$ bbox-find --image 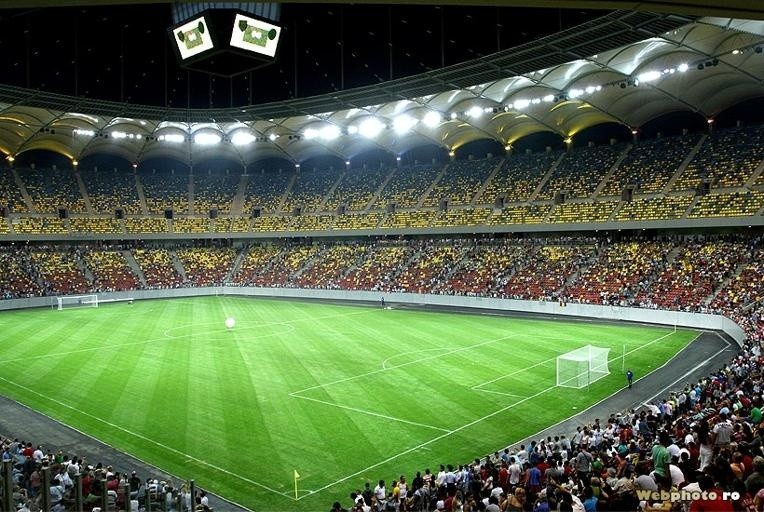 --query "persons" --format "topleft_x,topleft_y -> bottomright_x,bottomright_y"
0,124 -> 764,512
